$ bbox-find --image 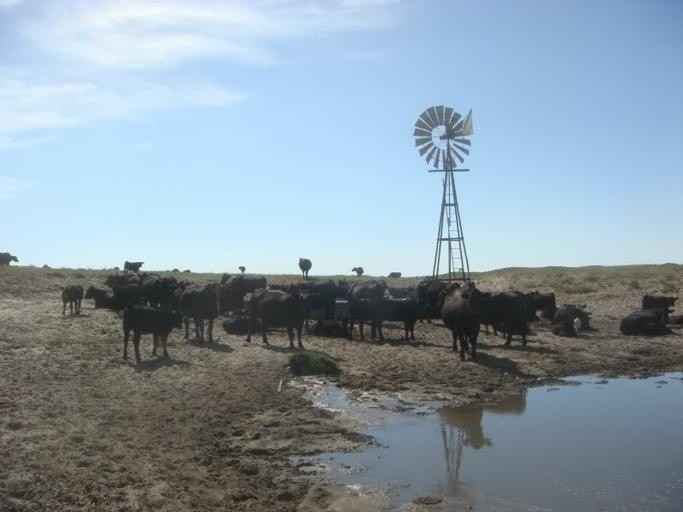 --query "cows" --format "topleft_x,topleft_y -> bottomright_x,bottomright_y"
83,272 -> 600,365
238,265 -> 245,273
299,257 -> 312,281
642,294 -> 679,311
124,261 -> 145,275
0,253 -> 19,266
619,308 -> 675,336
60,285 -> 84,315
352,267 -> 364,277
